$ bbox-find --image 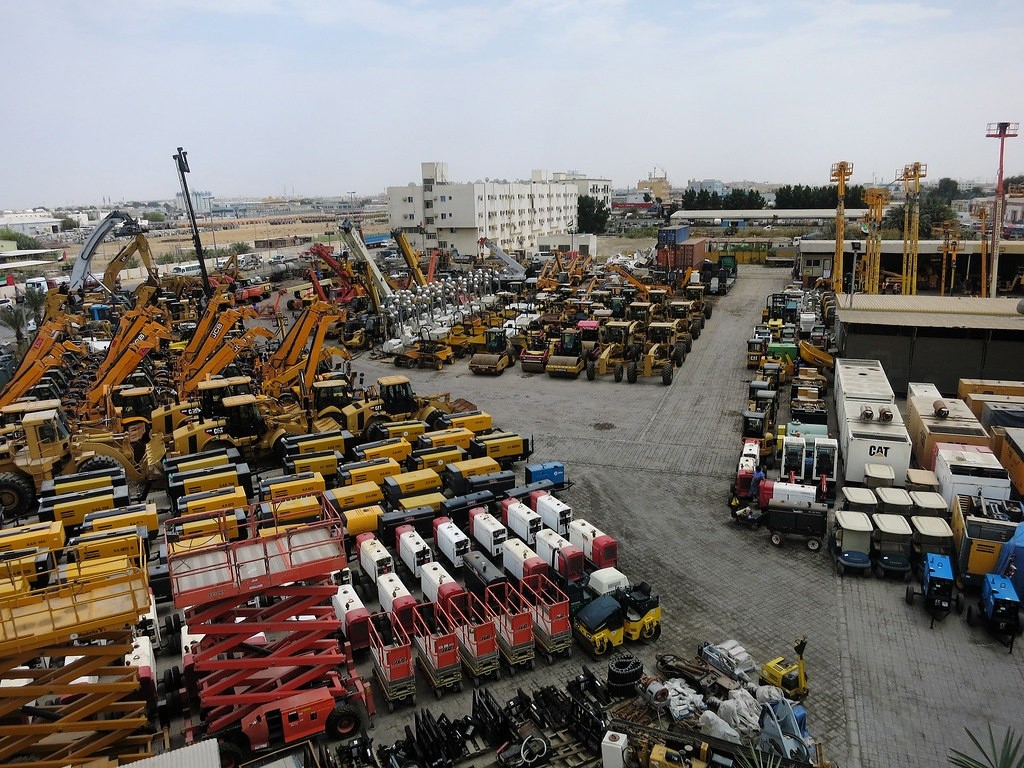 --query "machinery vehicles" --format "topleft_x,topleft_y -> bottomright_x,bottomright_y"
0,121 -> 1024,768
